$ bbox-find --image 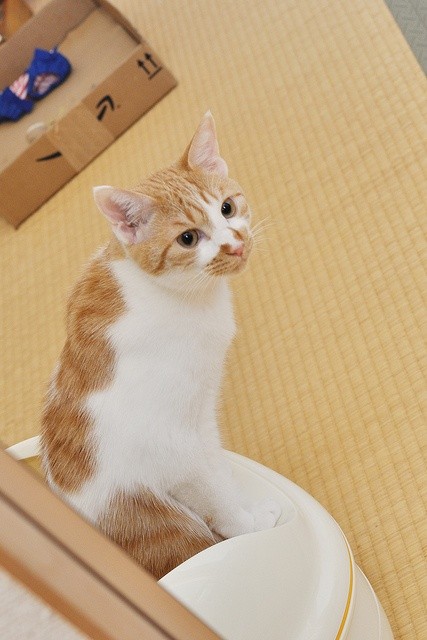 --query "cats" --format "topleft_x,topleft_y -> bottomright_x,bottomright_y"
39,107 -> 277,581
